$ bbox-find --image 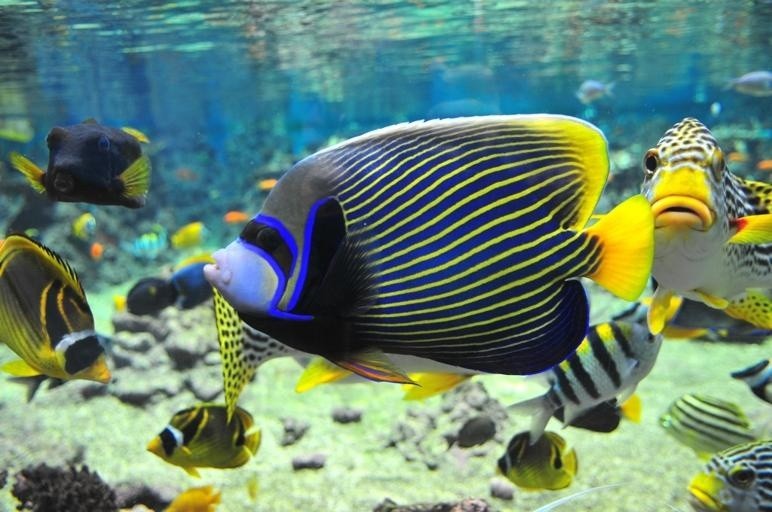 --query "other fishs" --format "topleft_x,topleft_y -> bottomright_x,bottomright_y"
0,69 -> 768,510
